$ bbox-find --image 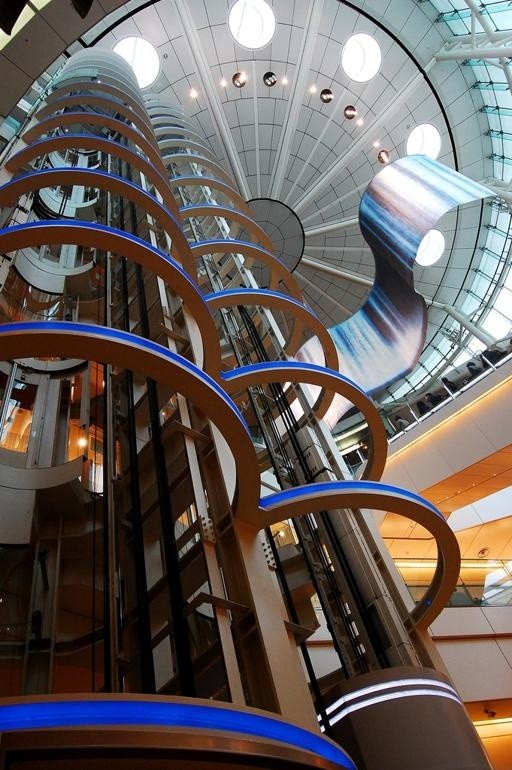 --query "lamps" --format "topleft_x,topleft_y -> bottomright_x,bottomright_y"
378,150 -> 390,163
232,72 -> 246,88
263,71 -> 277,87
343,105 -> 356,120
320,89 -> 333,103
